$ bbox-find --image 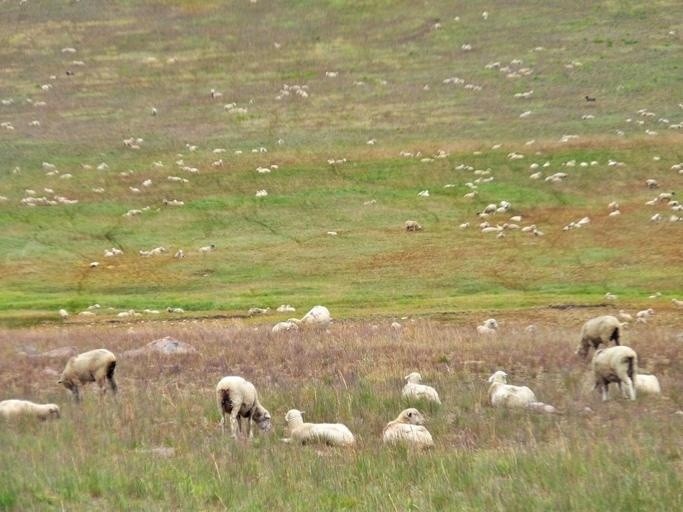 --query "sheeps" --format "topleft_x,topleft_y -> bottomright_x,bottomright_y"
0,10 -> 682,323
523,324 -> 537,334
214,375 -> 272,443
401,371 -> 442,408
0,394 -> 63,429
486,370 -> 553,414
475,319 -> 501,337
57,347 -> 120,405
279,408 -> 356,450
573,313 -> 623,362
588,343 -> 662,402
380,408 -> 434,451
